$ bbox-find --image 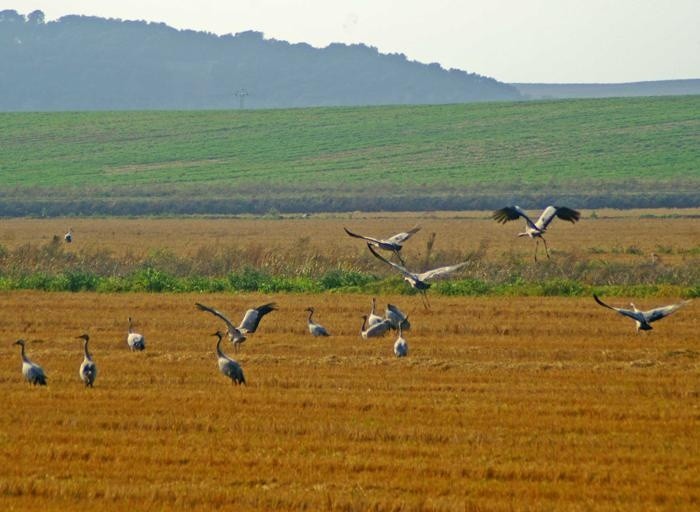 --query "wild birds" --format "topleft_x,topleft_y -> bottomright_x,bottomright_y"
127,317 -> 146,352
344,224 -> 422,266
493,205 -> 581,263
304,306 -> 332,338
361,298 -> 411,358
12,339 -> 48,386
593,293 -> 692,332
209,331 -> 247,387
75,334 -> 97,387
366,241 -> 471,310
194,301 -> 280,354
64,227 -> 73,242
